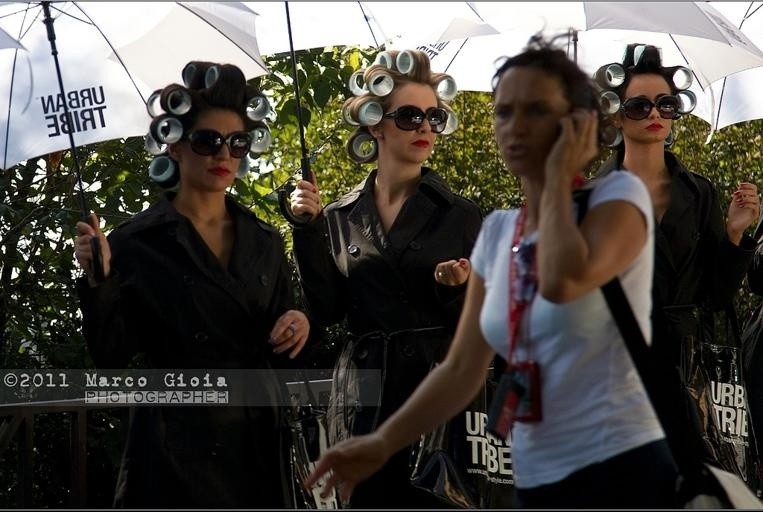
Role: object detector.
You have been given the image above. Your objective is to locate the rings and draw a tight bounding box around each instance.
[288,324,296,337]
[438,271,449,281]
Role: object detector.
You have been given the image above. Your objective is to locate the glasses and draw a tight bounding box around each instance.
[179,126,253,160]
[382,102,448,134]
[615,91,682,122]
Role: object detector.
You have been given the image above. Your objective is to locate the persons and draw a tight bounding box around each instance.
[72,62,309,509]
[305,48,679,508]
[290,50,484,509]
[591,59,759,377]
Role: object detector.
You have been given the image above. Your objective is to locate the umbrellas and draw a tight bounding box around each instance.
[234,0,501,227]
[0,0,269,293]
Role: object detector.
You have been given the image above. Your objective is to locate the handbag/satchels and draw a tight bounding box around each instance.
[405,278,515,509]
[676,462,763,510]
[672,288,763,495]
[276,341,342,509]
